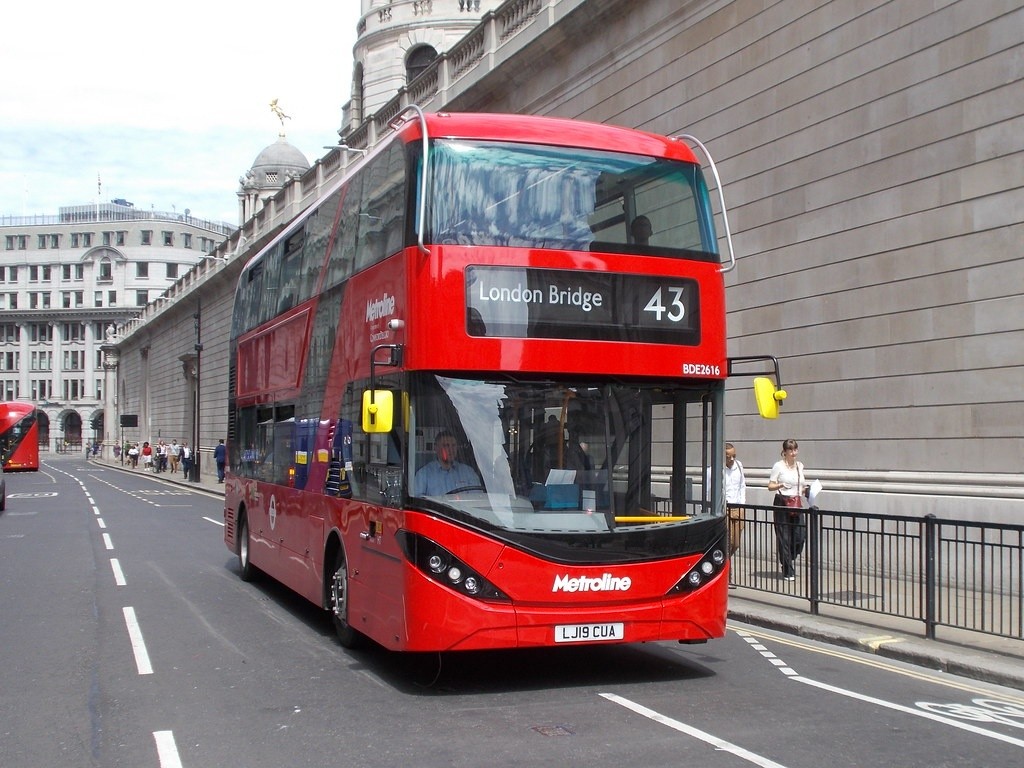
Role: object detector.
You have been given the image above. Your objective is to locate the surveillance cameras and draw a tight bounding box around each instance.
[388,319,398,331]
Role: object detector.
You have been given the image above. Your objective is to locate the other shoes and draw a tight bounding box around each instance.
[782,566,795,580]
[162,469,165,472]
[219,478,224,483]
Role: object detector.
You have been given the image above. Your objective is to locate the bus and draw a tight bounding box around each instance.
[218,103,789,657]
[0,402,39,472]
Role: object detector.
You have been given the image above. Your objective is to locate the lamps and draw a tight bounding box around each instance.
[198,256,227,264]
[129,318,144,323]
[323,145,367,156]
[154,296,174,303]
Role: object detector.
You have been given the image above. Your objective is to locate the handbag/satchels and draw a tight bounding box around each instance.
[782,495,803,517]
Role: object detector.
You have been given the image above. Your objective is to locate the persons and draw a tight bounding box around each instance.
[124,440,131,465]
[214,439,227,484]
[167,439,179,473]
[176,442,192,479]
[140,442,152,471]
[157,440,168,472]
[113,441,121,463]
[629,214,653,246]
[700,444,746,589]
[128,442,140,469]
[769,440,809,581]
[414,431,484,496]
[93,441,99,460]
